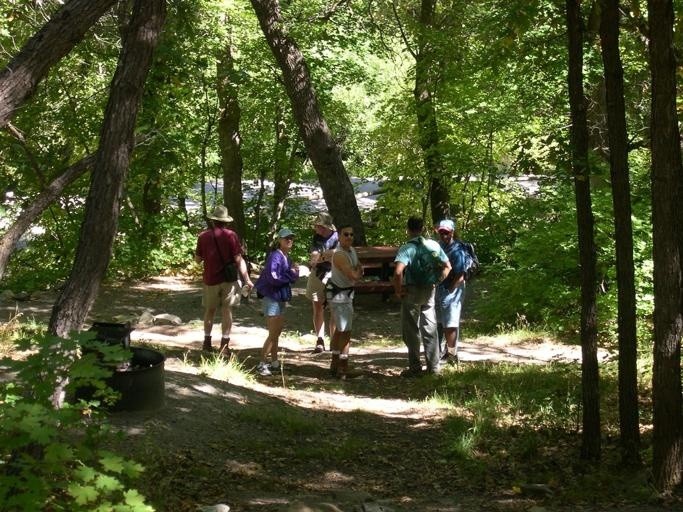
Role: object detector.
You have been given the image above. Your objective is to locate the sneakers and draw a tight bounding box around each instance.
[420,346,441,358]
[440,350,457,366]
[256,361,272,377]
[316,337,325,352]
[401,365,422,379]
[268,362,291,373]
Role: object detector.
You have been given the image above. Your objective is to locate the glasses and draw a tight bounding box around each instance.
[344,233,354,236]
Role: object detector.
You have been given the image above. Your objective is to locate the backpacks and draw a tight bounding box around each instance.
[406,237,448,287]
[458,240,480,280]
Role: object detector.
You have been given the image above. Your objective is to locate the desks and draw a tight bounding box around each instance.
[324,246,399,301]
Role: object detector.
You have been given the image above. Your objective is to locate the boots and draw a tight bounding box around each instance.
[336,357,364,378]
[202,336,213,350]
[330,353,354,374]
[219,337,231,356]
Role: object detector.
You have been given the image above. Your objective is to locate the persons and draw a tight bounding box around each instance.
[391,214,454,378]
[323,223,365,378]
[193,206,254,359]
[251,227,301,376]
[306,214,340,353]
[436,219,467,363]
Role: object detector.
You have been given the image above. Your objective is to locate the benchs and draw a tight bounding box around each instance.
[351,281,395,300]
[316,260,396,277]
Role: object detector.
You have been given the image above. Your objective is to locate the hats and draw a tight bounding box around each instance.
[438,220,455,234]
[278,229,296,237]
[206,206,234,224]
[309,212,338,232]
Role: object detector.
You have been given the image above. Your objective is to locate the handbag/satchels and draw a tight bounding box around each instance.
[224,255,251,283]
[280,271,292,302]
[310,246,325,279]
